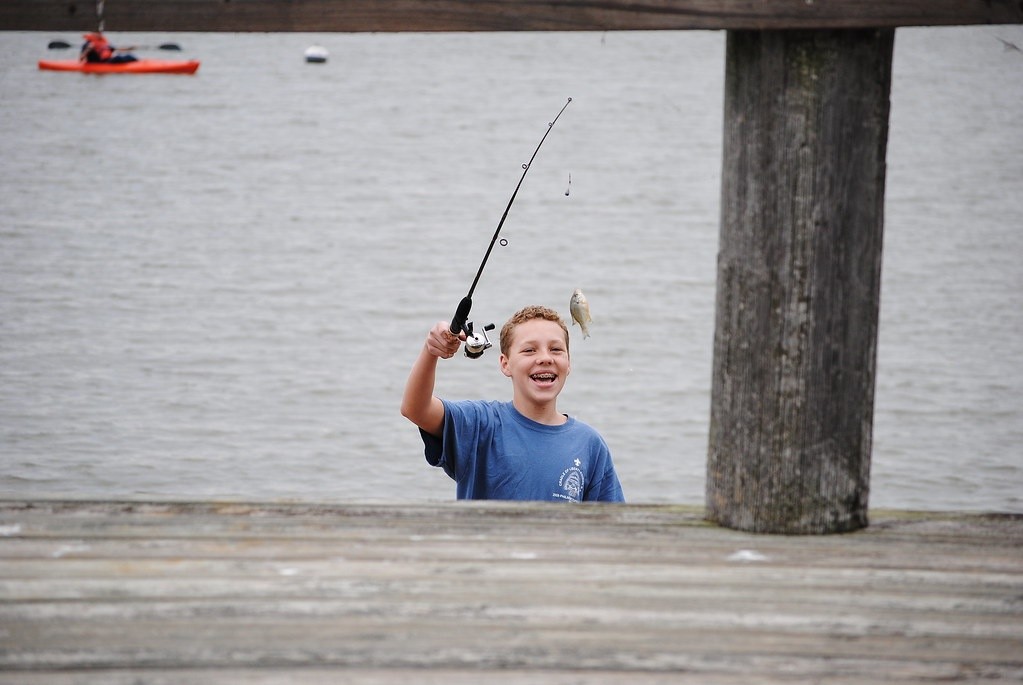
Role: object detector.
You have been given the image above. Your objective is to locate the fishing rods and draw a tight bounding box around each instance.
[449,95,572,360]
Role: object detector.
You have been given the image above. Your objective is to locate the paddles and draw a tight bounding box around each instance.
[46,42,183,51]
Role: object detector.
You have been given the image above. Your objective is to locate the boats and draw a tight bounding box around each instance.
[38,57,200,75]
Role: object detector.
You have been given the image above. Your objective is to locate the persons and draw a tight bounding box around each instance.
[401,306,628,504]
[80,25,138,63]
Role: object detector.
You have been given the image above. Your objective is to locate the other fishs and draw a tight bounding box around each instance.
[570,288,594,341]
